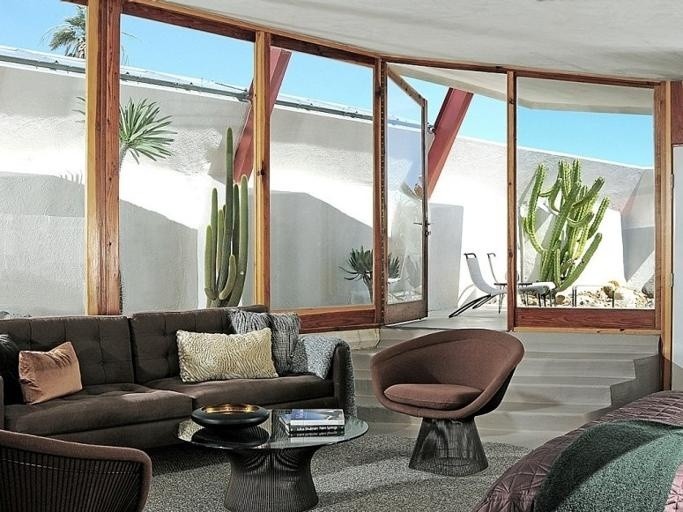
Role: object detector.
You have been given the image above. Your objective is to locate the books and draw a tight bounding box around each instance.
[279,414,345,437]
[290,409,345,431]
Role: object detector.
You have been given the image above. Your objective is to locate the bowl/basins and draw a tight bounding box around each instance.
[189,424,268,447]
[190,402,270,430]
